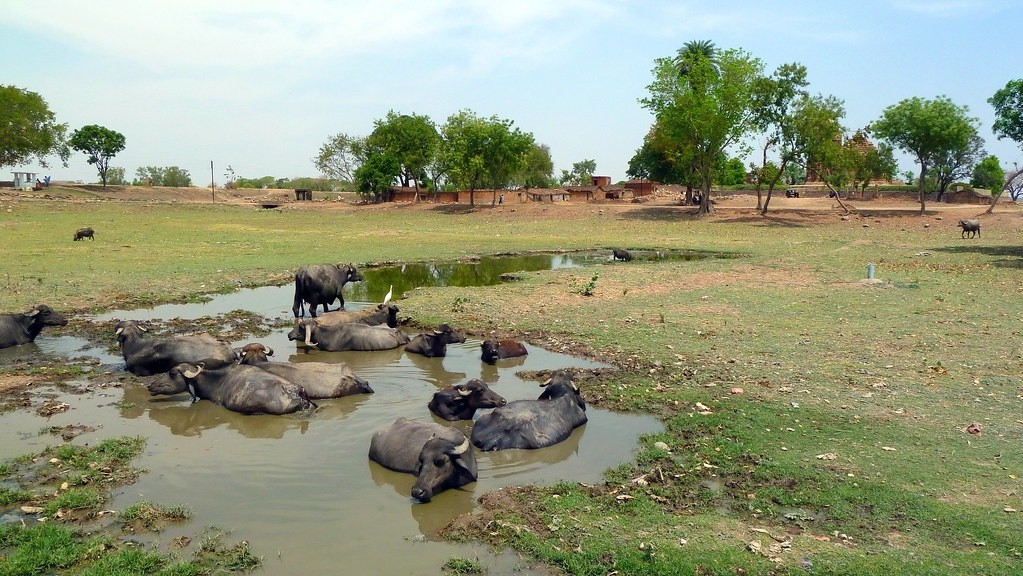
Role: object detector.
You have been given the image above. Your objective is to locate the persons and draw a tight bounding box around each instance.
[499,194,505,205]
[679,190,702,205]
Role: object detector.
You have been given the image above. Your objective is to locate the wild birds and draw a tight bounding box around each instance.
[384,284,393,304]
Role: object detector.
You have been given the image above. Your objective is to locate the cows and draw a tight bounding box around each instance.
[405,351,466,388]
[404,323,466,357]
[470,371,588,451]
[480,338,528,365]
[428,377,507,421]
[73,227,94,241]
[957,219,981,239]
[613,248,631,262]
[149,399,309,439]
[292,262,363,318]
[288,304,410,351]
[368,458,477,542]
[114,319,375,416]
[368,417,478,500]
[480,354,528,383]
[0,304,68,350]
